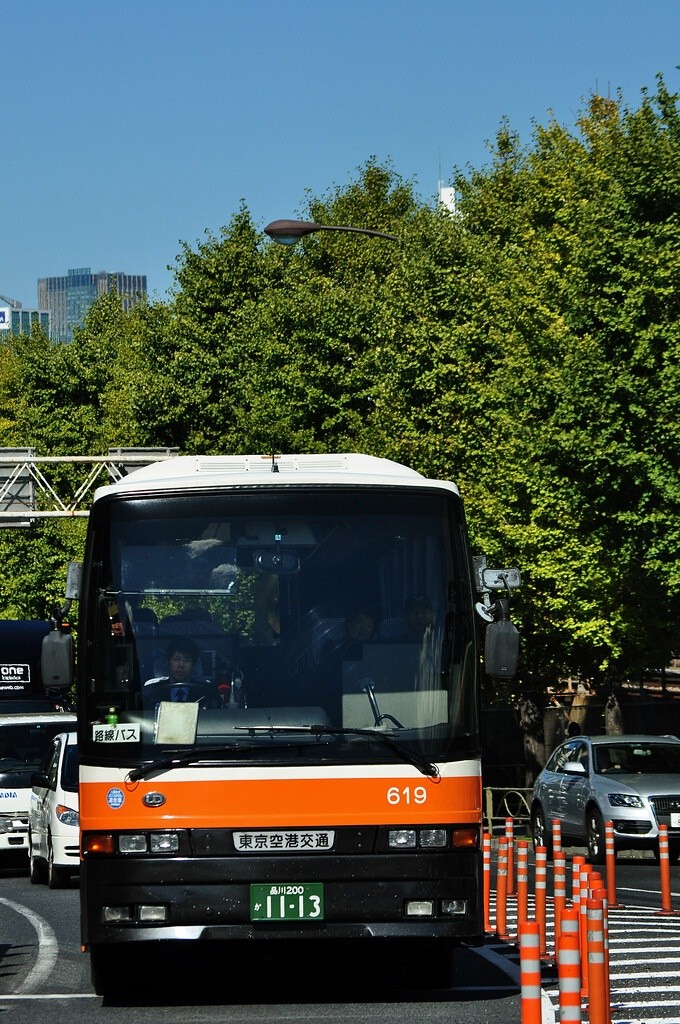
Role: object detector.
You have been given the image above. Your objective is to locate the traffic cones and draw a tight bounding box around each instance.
[482,818,617,1024]
[604,822,623,909]
[654,825,679,914]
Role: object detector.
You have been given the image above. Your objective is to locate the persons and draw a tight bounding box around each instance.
[147,634,223,713]
[404,592,433,636]
[345,609,374,642]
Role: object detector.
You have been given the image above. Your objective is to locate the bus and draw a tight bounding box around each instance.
[67,451,519,1006]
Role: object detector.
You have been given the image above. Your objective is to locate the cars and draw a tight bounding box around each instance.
[530,734,680,865]
[27,732,80,889]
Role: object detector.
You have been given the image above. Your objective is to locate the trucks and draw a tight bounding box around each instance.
[0,711,77,876]
